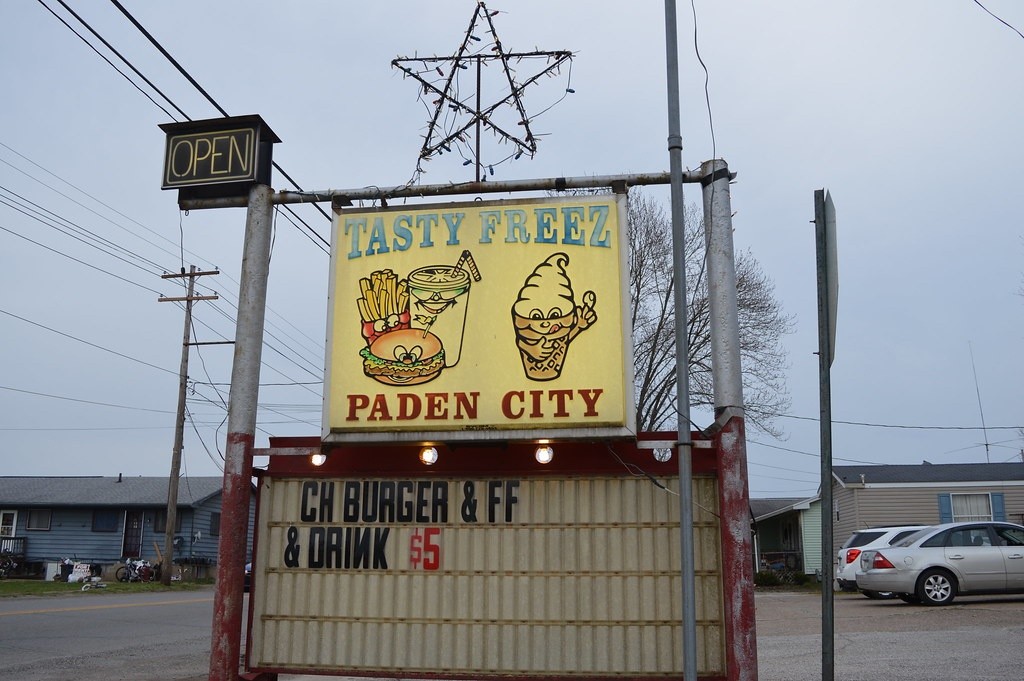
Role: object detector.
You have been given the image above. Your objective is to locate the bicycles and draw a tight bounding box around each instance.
[116,556,156,582]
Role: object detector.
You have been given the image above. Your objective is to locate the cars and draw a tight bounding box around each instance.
[855,521,1024,606]
[243,563,251,593]
[837,522,991,599]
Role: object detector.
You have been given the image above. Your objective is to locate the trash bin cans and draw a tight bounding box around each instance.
[60,564,74,582]
[89,564,102,576]
